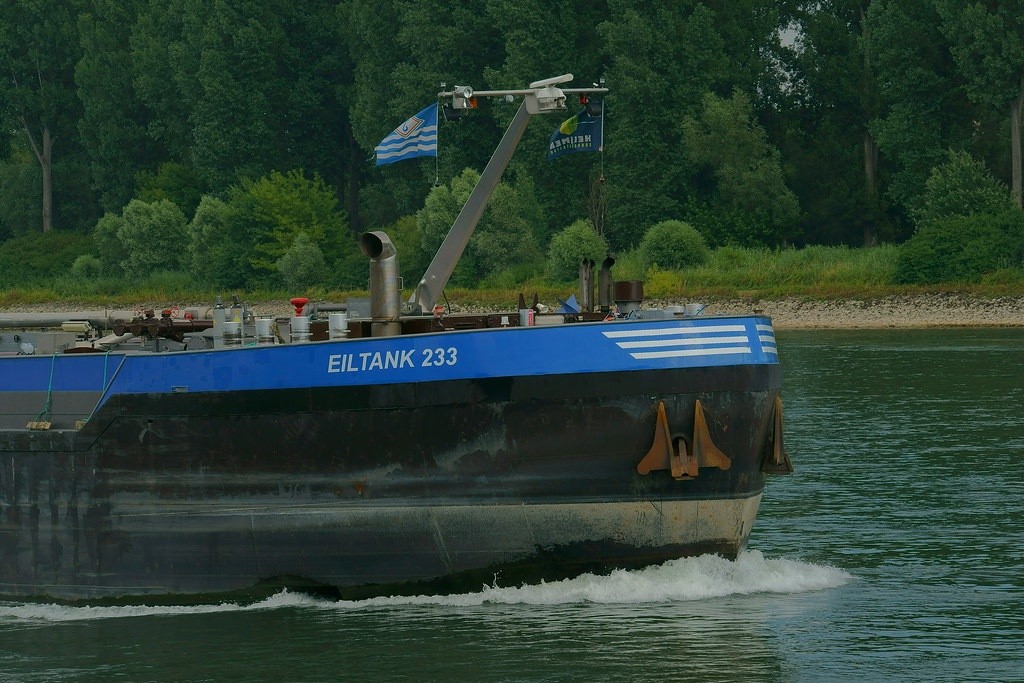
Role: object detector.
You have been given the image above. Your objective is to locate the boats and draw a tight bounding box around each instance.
[0,72,793,607]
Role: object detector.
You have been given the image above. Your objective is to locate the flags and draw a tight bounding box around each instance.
[549,98,602,161]
[374,103,437,165]
